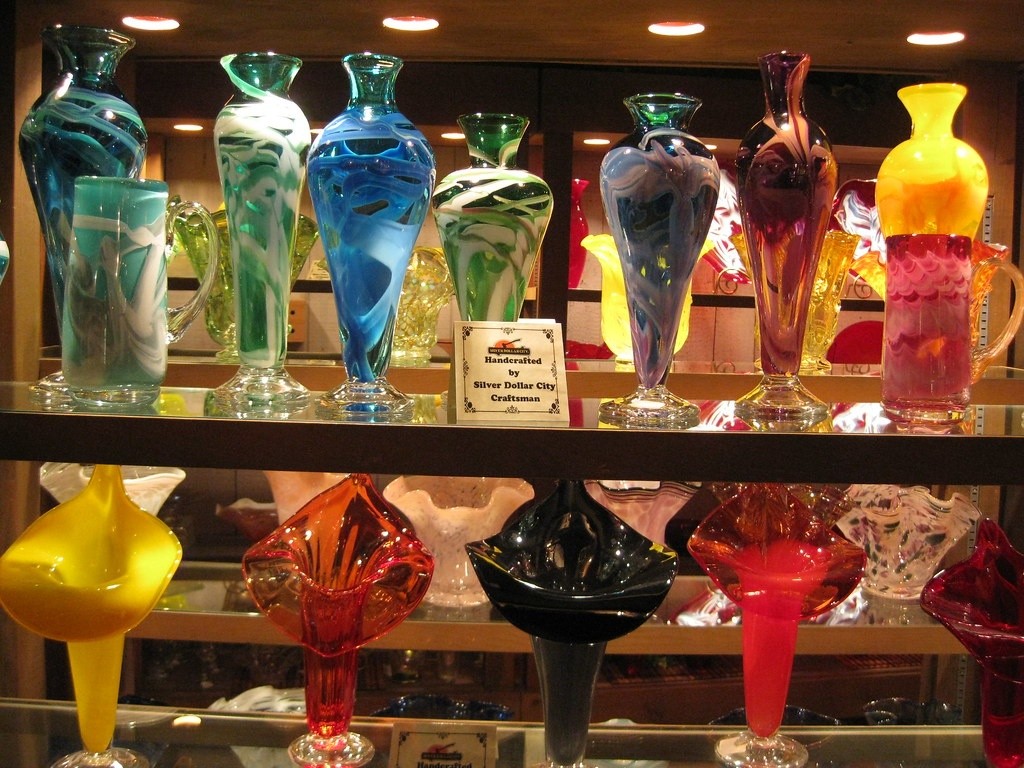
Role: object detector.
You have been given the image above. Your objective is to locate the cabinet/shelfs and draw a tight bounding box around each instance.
[0,380,1024,766]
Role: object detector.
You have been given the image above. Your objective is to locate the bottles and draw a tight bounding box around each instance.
[734,52,838,418]
[212,51,313,405]
[432,112,553,323]
[873,83,990,239]
[599,92,720,429]
[307,52,436,415]
[567,176,593,292]
[18,23,147,399]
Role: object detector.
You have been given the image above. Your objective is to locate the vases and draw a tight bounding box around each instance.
[19,22,989,430]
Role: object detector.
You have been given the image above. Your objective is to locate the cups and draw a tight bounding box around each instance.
[314,241,456,370]
[730,223,864,377]
[61,176,219,409]
[581,225,713,365]
[881,234,1024,426]
[165,194,320,364]
